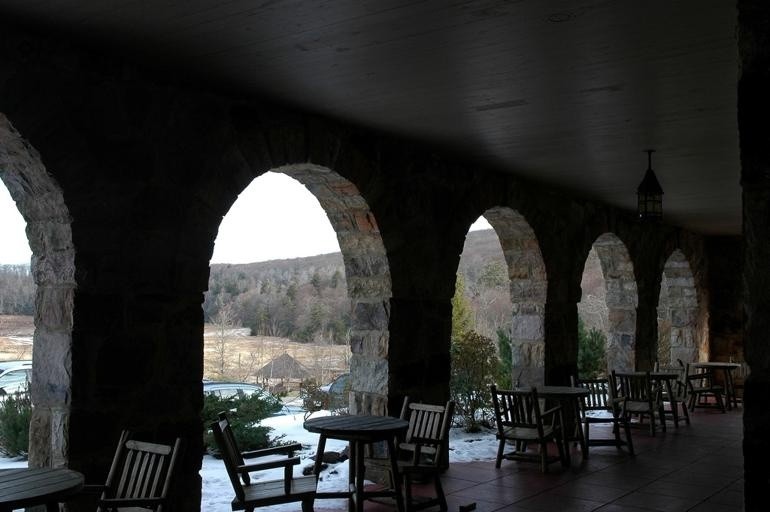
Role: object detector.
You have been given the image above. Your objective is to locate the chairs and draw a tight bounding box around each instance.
[358,395,456,512]
[490,354,752,475]
[74,425,182,512]
[213,411,319,511]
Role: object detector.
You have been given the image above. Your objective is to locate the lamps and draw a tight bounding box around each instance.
[635,150,665,220]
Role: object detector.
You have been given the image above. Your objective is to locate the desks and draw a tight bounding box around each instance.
[303,413,411,512]
[0,465,85,511]
